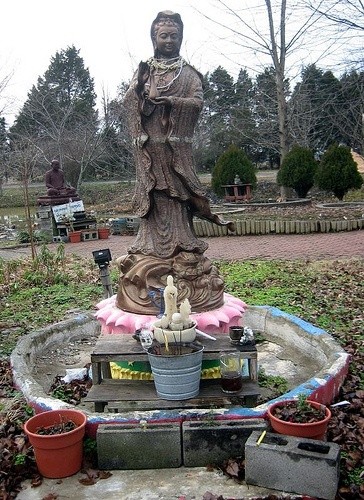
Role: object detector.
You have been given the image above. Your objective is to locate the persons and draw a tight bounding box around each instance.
[44,159,76,195]
[163,274,178,319]
[114,9,236,259]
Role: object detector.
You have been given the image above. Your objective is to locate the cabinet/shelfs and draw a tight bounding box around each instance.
[83,334,261,413]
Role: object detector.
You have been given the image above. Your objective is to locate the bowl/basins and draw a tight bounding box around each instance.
[153,319,198,344]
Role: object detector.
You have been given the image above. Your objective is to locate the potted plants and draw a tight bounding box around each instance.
[56,198,82,243]
[88,200,110,239]
[267,393,332,440]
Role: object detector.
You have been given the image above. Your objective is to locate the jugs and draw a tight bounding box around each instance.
[218,353,243,394]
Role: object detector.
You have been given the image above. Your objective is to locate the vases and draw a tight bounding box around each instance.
[24,409,87,479]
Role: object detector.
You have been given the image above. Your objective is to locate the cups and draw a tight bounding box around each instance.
[229,325,244,344]
[140,331,154,351]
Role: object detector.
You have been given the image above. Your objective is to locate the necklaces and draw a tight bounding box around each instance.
[143,56,187,93]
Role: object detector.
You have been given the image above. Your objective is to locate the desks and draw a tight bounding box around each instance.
[221,184,252,202]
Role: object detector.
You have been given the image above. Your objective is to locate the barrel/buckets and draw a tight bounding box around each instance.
[145,342,205,401]
